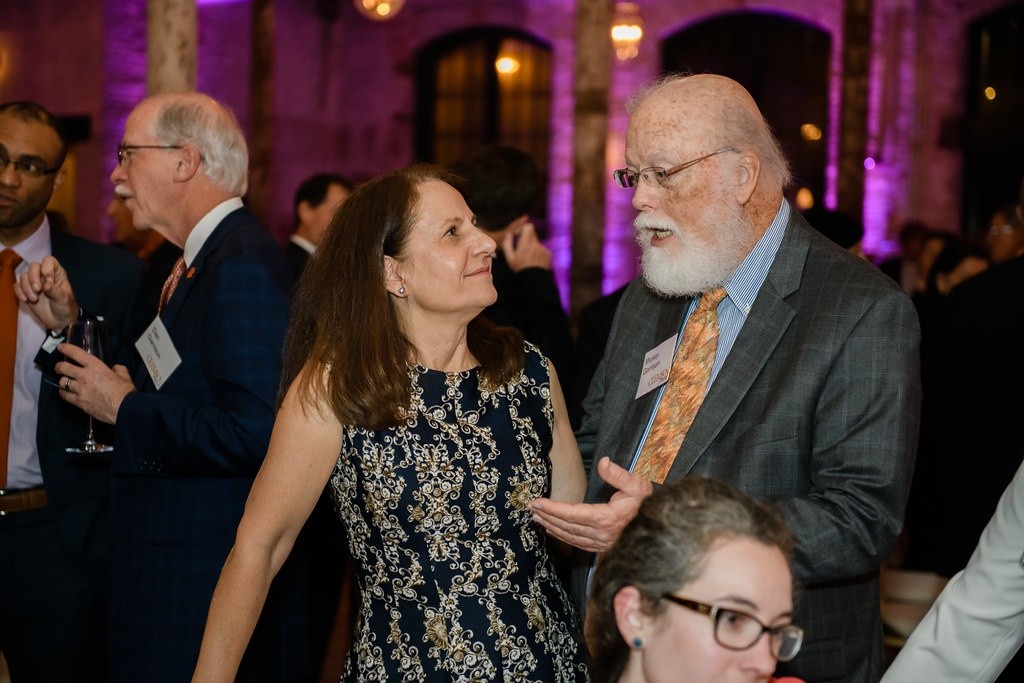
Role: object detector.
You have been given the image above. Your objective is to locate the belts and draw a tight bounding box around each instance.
[0,490,49,516]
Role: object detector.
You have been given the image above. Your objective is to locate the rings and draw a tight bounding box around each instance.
[65,379,71,391]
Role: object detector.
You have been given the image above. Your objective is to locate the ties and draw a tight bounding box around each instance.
[156,257,188,319]
[0,249,23,488]
[593,285,728,567]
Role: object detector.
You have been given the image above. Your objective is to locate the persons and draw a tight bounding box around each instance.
[527,74,920,683]
[817,192,1024,683]
[0,90,368,683]
[191,161,590,683]
[583,478,805,683]
[453,148,631,445]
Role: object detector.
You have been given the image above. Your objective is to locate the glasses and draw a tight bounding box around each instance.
[612,146,743,189]
[659,591,803,662]
[0,157,57,178]
[117,143,204,166]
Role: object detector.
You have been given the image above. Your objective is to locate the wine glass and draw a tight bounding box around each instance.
[61,318,115,453]
